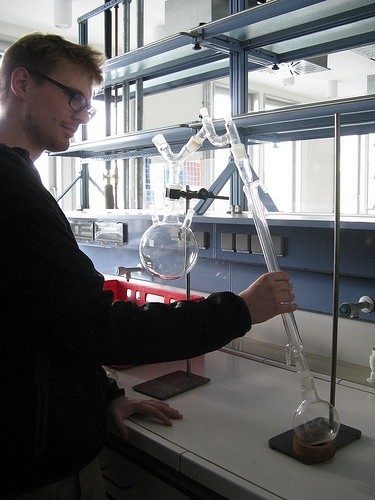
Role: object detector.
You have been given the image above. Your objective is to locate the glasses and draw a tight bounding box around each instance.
[31,71,96,119]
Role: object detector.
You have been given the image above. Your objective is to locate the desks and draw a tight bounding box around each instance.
[102,272,375,500]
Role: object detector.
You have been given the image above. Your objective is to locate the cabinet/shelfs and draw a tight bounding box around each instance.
[48,0,375,230]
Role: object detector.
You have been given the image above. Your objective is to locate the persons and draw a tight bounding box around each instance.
[0,31,298,500]
